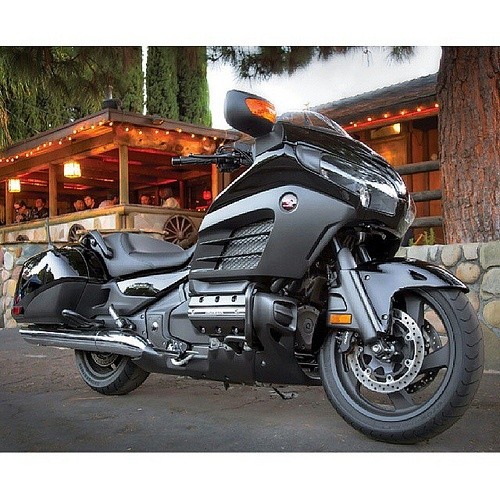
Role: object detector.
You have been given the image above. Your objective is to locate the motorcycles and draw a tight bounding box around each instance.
[11,90,484,444]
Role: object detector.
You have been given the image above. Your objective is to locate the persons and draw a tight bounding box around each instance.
[73,199,84,211]
[99,193,119,207]
[159,188,180,209]
[31,198,49,220]
[84,195,98,209]
[14,200,33,222]
[140,193,152,205]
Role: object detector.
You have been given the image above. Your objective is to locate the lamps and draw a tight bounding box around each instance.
[63,162,81,179]
[8,177,21,192]
[150,115,165,125]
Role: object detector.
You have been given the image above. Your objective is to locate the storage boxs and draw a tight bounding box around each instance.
[11,247,109,325]
